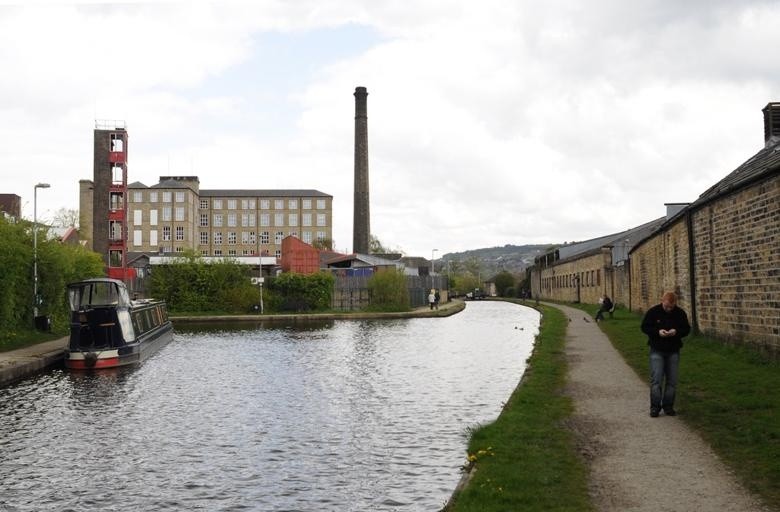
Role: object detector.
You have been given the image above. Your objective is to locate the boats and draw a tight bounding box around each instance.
[63,278,175,372]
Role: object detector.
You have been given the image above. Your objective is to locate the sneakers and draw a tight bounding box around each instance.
[664,409,675,416]
[650,409,659,417]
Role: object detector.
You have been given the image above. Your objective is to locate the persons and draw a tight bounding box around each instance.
[640,290,691,418]
[84,304,100,346]
[595,293,613,322]
[434,290,440,310]
[427,291,436,310]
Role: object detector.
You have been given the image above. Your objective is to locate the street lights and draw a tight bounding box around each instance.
[431,249,439,288]
[478,272,483,284]
[31,182,50,320]
[447,260,453,290]
[258,234,269,314]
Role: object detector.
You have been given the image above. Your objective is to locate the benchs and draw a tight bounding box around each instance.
[608,303,616,318]
